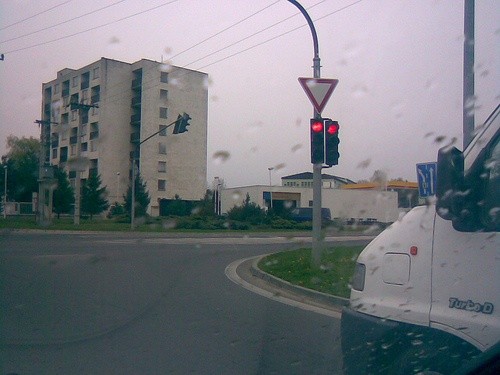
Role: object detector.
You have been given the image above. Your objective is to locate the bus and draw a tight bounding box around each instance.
[340,101,499,374]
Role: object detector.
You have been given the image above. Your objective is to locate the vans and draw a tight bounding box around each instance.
[288,208,331,223]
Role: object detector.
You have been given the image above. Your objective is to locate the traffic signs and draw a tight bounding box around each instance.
[416,162,438,199]
[298,77,339,114]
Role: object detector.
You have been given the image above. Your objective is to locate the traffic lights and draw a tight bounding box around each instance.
[310,119,324,163]
[325,121,338,165]
[179,112,192,133]
[173,113,183,134]
